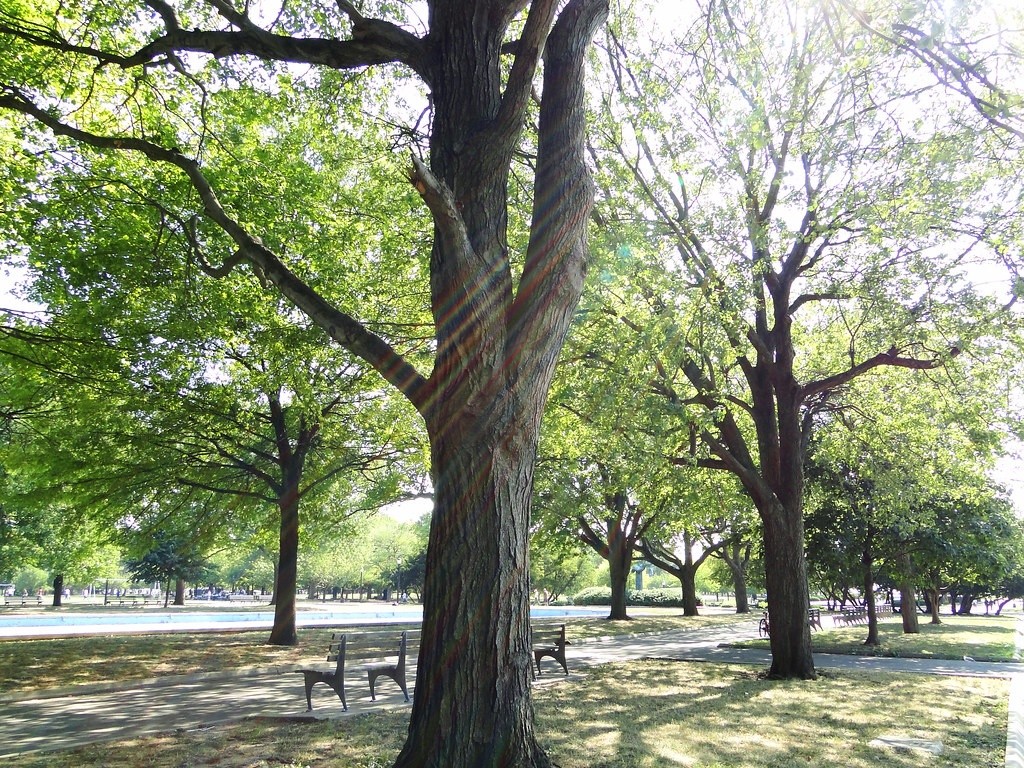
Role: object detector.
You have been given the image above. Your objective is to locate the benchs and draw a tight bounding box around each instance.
[294,629,421,712]
[759,608,822,637]
[532,624,570,677]
[876,604,893,621]
[833,606,869,628]
